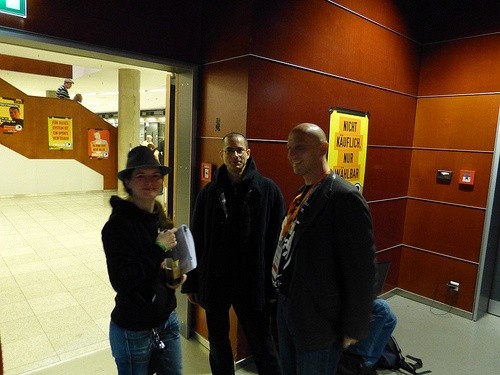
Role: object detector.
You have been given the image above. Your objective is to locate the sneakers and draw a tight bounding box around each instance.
[339,348,377,375]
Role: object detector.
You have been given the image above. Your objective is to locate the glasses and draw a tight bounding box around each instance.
[222,148,247,153]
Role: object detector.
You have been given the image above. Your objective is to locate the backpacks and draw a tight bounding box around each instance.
[374,334,432,375]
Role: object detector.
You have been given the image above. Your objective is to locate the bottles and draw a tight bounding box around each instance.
[164,230,181,283]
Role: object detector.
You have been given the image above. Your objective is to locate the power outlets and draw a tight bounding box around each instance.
[449,281,460,292]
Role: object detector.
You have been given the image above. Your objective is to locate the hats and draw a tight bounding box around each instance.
[116,146,170,182]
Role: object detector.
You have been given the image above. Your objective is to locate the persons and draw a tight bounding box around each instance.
[142,133,166,195]
[56,79,83,105]
[102,146,187,374]
[268,123,376,375]
[0,107,25,131]
[180,132,285,375]
[339,299,397,375]
